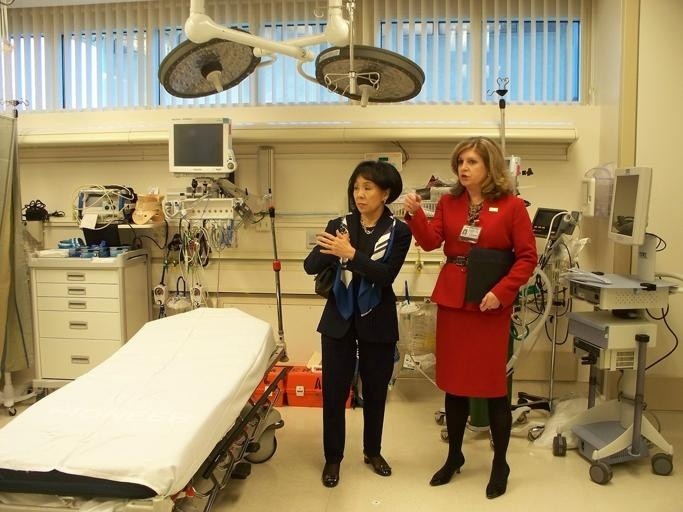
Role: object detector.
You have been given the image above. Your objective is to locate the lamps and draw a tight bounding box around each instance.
[157,0,422,107]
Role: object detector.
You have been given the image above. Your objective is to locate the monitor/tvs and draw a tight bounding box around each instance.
[606,166,653,246]
[168,117,237,175]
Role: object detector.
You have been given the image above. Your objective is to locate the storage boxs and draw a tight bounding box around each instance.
[250,365,355,410]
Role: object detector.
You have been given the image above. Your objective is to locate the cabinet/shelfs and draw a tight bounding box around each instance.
[28,246,149,403]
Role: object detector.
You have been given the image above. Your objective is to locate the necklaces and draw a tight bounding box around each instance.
[360,216,374,235]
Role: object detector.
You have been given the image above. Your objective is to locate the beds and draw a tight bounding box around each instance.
[2,307,283,511]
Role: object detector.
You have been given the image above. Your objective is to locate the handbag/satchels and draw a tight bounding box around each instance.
[464,246,521,306]
[315,263,337,299]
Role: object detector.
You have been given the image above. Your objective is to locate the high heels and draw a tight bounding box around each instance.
[486,458,510,498]
[430,453,465,486]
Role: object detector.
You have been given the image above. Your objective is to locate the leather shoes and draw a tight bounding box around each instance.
[322,459,340,487]
[364,454,391,477]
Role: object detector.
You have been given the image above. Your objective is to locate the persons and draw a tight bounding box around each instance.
[301,160,413,488]
[401,134,539,501]
